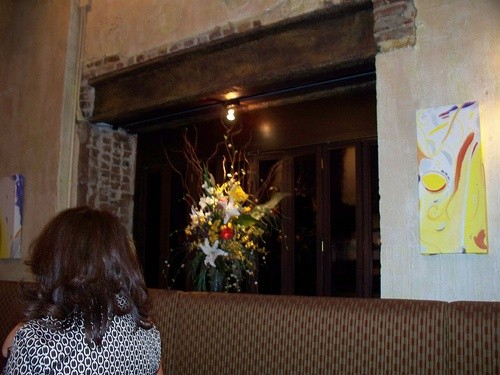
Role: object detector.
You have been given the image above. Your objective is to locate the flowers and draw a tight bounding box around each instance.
[162,117,304,295]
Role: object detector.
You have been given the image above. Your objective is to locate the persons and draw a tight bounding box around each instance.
[0,207,167,375]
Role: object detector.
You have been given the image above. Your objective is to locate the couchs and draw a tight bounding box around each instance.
[0,280,500,375]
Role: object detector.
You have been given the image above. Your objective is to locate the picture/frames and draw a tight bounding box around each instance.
[0,175,23,261]
[415,101,489,256]
